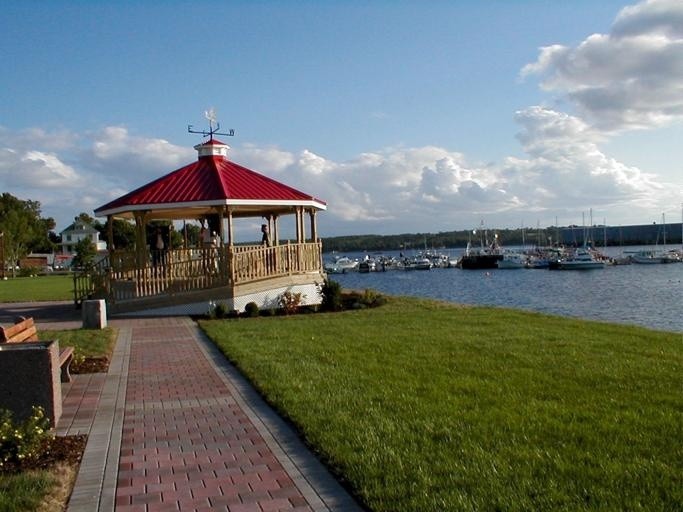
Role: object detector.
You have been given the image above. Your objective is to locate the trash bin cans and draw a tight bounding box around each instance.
[83,299,106,329]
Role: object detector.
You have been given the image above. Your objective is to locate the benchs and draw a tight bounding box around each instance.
[0,315,75,382]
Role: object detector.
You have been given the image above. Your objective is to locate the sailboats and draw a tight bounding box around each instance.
[458,207,683,271]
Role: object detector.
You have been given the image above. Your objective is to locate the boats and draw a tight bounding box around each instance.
[323,250,458,274]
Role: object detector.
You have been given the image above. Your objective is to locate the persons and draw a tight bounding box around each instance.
[150,224,272,278]
